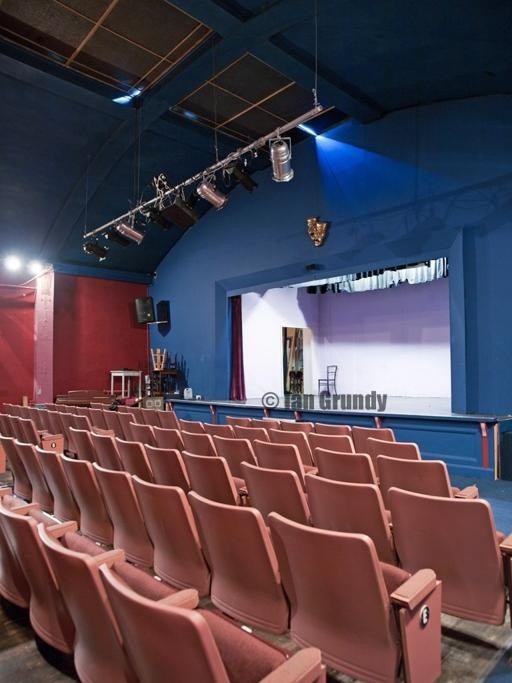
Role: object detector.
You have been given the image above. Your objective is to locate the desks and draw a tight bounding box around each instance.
[108,369,144,397]
[152,370,179,394]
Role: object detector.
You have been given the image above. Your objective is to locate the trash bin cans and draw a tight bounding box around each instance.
[142,397,164,411]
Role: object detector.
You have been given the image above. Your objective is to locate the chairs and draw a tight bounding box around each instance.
[0,394,512,682]
[316,364,338,395]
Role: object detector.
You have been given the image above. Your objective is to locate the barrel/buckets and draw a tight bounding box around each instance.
[183,387,192,400]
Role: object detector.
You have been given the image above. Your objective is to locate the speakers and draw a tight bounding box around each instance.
[135,296,155,323]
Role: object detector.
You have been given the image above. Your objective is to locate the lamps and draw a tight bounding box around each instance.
[79,0,325,261]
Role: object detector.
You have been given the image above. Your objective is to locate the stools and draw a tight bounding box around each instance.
[147,347,169,370]
